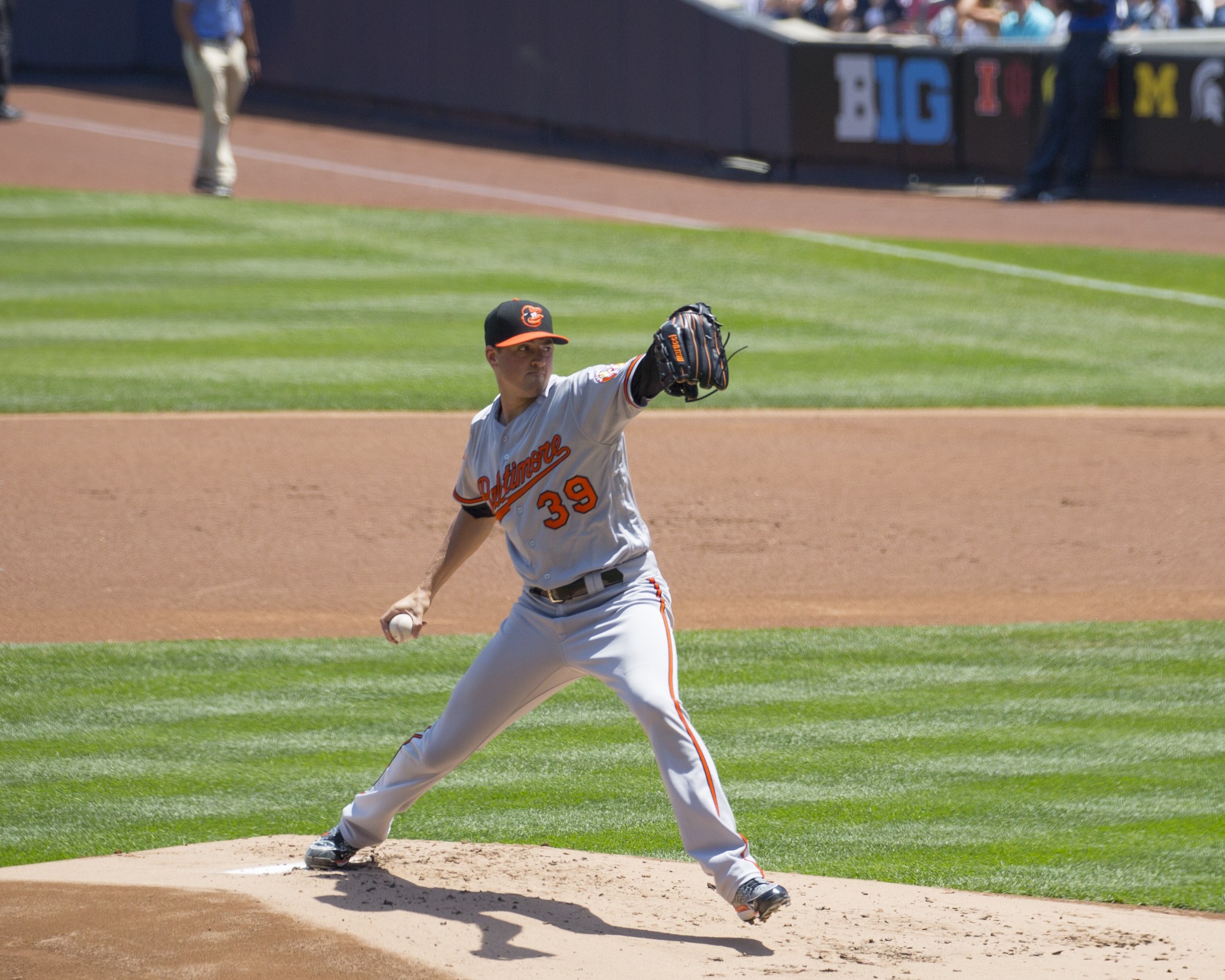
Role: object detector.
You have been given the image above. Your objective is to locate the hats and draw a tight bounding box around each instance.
[484,298,569,347]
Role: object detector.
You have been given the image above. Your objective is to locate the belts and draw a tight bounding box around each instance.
[529,568,623,604]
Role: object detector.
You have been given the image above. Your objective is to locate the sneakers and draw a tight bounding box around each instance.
[732,877,791,924]
[304,822,359,871]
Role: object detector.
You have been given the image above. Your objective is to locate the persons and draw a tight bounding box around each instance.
[173,0,261,198]
[304,300,792,924]
[744,0,1225,202]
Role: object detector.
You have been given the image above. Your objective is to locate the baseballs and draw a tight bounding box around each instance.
[388,613,413,643]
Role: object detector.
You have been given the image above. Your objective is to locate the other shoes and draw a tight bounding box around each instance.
[213,185,232,198]
[195,178,215,191]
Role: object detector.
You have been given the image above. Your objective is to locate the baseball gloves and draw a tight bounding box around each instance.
[652,303,730,402]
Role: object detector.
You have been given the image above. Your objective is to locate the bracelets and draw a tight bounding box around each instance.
[248,53,260,60]
